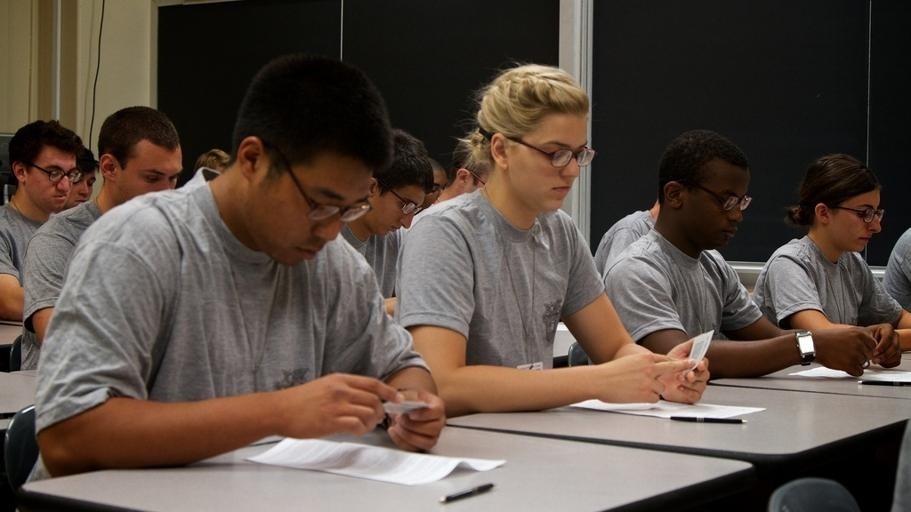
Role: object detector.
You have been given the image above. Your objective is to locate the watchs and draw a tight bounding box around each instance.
[794,329,817,366]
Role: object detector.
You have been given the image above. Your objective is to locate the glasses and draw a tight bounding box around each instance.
[31,164,82,183]
[689,183,751,210]
[390,189,423,215]
[280,155,373,224]
[831,206,885,224]
[502,134,596,167]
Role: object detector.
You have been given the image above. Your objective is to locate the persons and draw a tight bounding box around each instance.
[752,154,911,357]
[2,105,493,367]
[400,63,710,418]
[588,198,662,366]
[602,129,878,377]
[32,54,448,475]
[883,228,911,312]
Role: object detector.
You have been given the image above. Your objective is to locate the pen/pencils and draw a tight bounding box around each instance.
[671,416,748,424]
[442,483,495,502]
[858,379,910,387]
